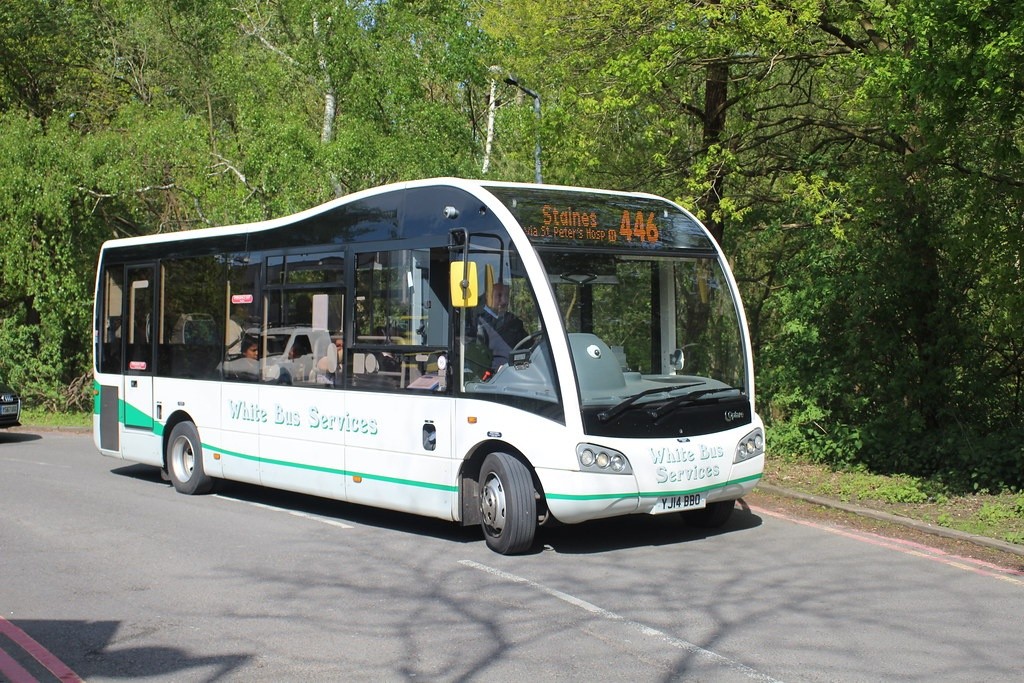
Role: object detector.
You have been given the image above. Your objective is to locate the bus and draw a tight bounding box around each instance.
[91,177,765,556]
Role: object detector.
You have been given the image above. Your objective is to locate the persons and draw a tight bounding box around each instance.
[240,332,258,359]
[476,283,534,373]
[331,332,344,351]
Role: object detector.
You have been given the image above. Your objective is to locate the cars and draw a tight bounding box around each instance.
[0,381,22,428]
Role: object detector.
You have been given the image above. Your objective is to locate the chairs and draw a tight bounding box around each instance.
[455,305,479,338]
[146,310,222,347]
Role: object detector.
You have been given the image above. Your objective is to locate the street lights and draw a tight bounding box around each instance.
[504,74,544,184]
